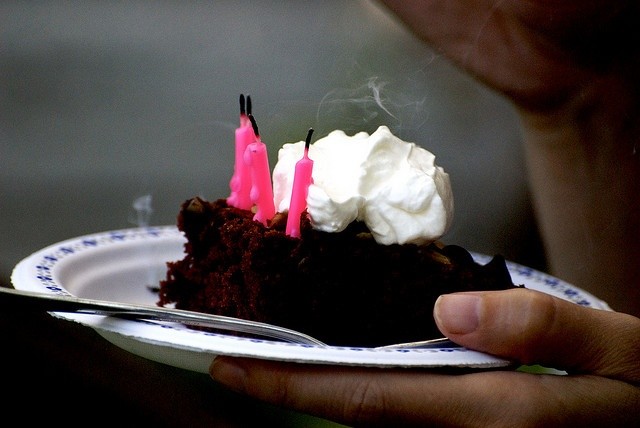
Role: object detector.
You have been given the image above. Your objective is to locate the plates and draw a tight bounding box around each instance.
[9,226,614,379]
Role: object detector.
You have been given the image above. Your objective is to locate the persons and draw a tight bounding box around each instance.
[208,1,640,428]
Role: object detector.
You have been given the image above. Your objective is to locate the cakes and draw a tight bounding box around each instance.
[151,125,526,349]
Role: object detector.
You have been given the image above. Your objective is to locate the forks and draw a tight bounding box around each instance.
[0,284,322,343]
